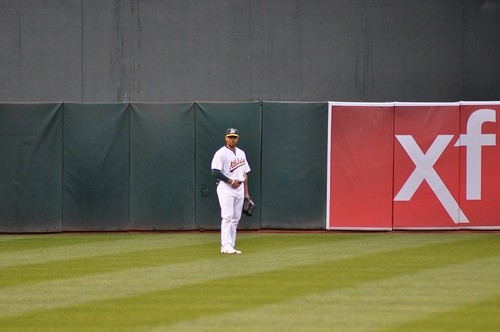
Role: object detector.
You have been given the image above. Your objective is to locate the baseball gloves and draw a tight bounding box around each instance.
[242,198,256,217]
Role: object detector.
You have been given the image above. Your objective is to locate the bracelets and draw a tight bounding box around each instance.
[230,180,234,186]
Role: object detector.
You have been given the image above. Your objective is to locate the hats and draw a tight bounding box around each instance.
[226,128,238,136]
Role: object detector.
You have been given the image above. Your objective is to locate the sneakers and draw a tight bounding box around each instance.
[221,244,241,254]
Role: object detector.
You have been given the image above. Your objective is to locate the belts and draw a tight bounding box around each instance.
[240,181,245,183]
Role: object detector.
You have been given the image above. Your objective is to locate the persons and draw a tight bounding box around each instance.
[210,127,251,254]
